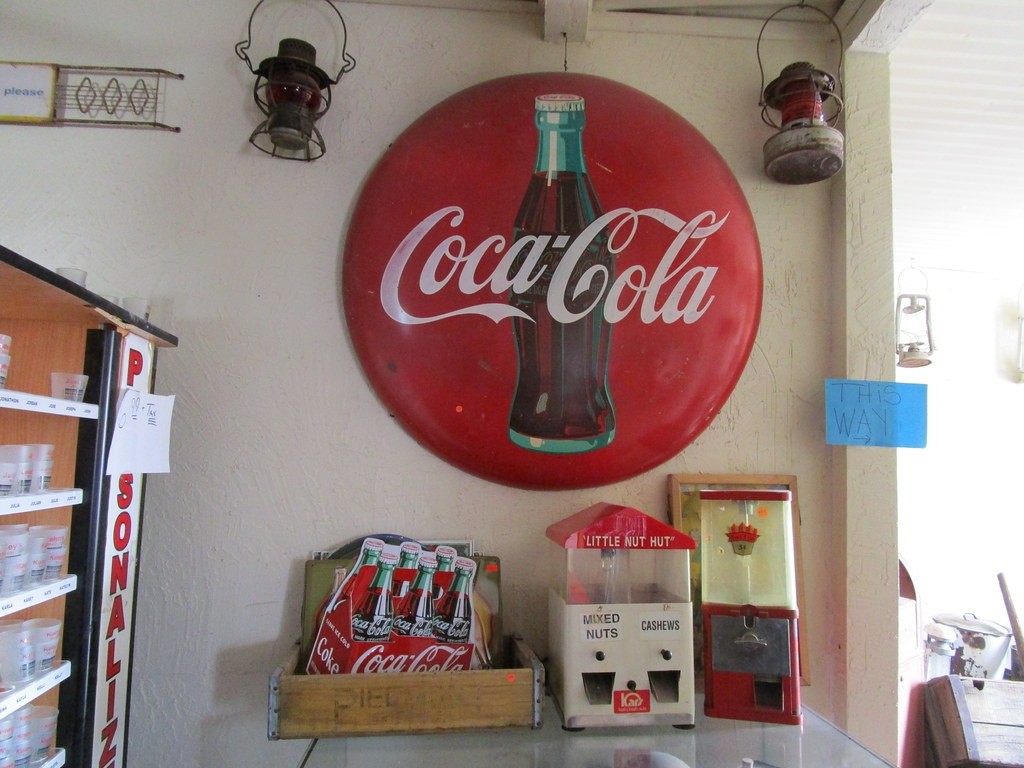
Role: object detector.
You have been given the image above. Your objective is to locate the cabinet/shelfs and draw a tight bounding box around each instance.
[0,245,179,768]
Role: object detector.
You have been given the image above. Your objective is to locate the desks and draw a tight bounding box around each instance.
[295,687,897,768]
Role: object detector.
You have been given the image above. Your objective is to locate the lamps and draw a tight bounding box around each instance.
[756,4,845,184]
[895,256,935,368]
[234,0,356,163]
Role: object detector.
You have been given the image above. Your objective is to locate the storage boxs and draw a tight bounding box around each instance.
[266,635,543,740]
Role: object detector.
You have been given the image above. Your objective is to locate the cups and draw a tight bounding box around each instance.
[1,354,12,388]
[51,370,88,402]
[57,267,153,322]
[1,334,13,355]
[0,443,59,768]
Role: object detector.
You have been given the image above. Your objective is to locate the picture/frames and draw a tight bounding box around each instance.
[666,473,811,694]
